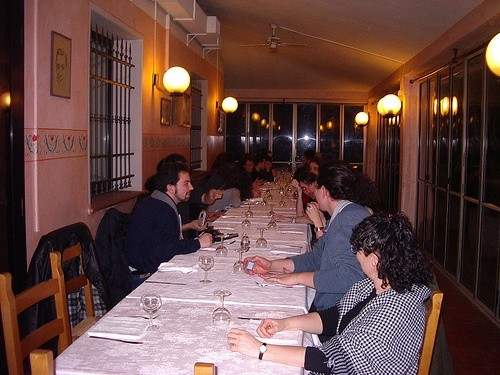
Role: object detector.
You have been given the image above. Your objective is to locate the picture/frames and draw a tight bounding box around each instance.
[182,94,192,127]
[161,98,170,125]
[51,30,73,98]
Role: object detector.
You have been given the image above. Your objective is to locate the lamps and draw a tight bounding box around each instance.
[486,32,500,76]
[163,66,190,95]
[219,96,238,113]
[354,111,369,126]
[377,94,402,118]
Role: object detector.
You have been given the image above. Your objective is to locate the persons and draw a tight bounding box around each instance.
[291,148,331,241]
[165,154,222,238]
[226,211,434,375]
[243,158,374,311]
[199,150,273,214]
[122,158,213,290]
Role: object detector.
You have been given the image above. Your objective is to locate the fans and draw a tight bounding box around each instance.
[238,23,308,49]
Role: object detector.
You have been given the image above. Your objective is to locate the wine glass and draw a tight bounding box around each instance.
[242,213,252,229]
[141,293,162,331]
[245,199,254,217]
[198,255,214,284]
[267,212,278,231]
[256,227,267,248]
[263,171,298,206]
[266,203,276,219]
[211,290,233,328]
[233,248,246,274]
[215,235,228,257]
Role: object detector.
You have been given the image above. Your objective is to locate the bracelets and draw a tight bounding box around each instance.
[313,226,325,232]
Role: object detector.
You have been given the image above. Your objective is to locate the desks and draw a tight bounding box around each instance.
[51,181,324,375]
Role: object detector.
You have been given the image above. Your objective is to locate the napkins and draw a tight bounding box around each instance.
[201,244,219,250]
[213,223,237,230]
[113,316,164,327]
[158,262,197,274]
[279,227,304,234]
[270,245,302,255]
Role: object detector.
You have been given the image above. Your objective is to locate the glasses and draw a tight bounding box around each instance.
[351,244,363,255]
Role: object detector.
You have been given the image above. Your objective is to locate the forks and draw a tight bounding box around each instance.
[114,314,160,320]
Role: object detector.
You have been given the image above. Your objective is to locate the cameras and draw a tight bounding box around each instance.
[198,211,207,226]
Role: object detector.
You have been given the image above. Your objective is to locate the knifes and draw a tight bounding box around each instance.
[145,280,187,286]
[89,335,143,345]
[237,316,265,321]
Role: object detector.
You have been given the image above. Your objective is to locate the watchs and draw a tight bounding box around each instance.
[258,343,267,360]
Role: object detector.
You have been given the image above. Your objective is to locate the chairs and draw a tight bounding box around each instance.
[94,208,130,306]
[416,270,444,375]
[22,222,113,344]
[0,251,71,375]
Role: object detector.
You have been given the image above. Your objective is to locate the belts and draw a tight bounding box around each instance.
[132,273,151,279]
